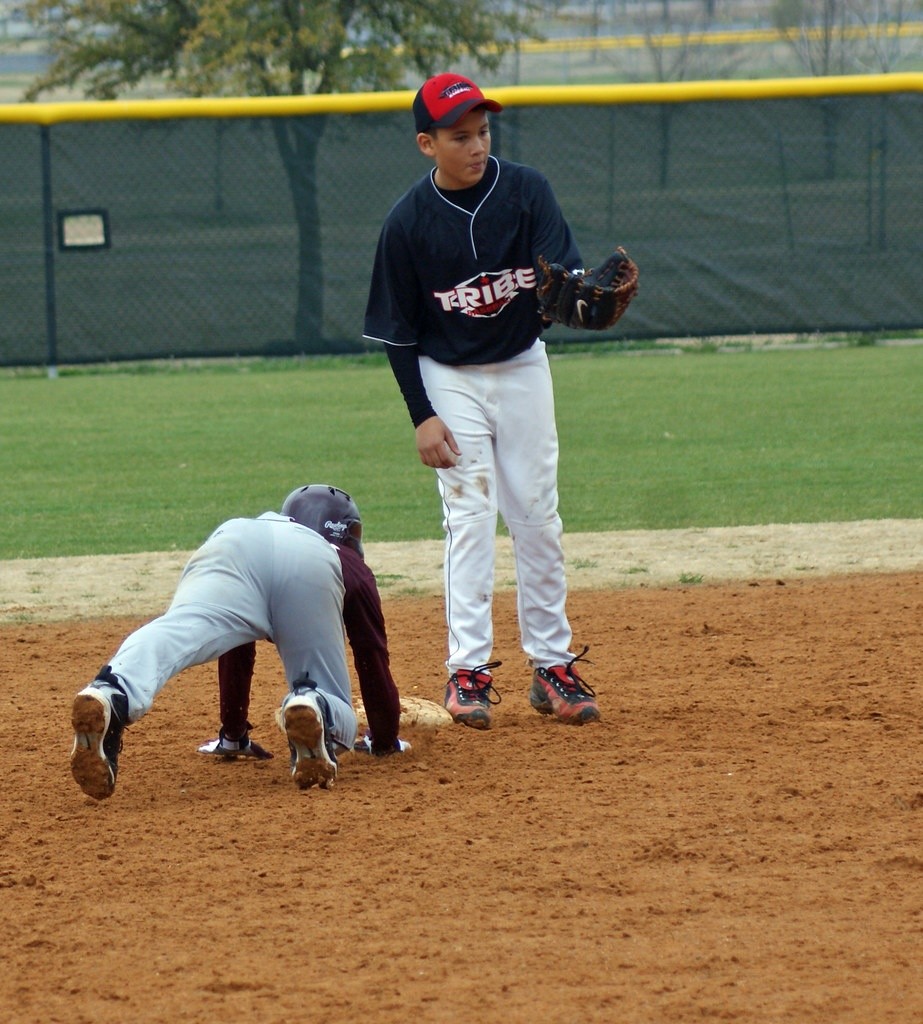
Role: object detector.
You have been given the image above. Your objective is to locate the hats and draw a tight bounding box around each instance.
[413,74,503,134]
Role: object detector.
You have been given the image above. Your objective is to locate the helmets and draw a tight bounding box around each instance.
[281,484,366,555]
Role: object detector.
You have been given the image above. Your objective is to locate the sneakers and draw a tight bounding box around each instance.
[68,665,130,800]
[530,644,600,724]
[444,662,501,728]
[282,679,338,791]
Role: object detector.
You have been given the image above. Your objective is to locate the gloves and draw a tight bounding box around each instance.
[198,737,273,759]
[356,736,412,754]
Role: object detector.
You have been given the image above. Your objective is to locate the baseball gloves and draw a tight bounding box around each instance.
[534,242,642,333]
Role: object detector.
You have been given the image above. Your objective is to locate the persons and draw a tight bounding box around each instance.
[70,485,413,800]
[362,74,639,728]
